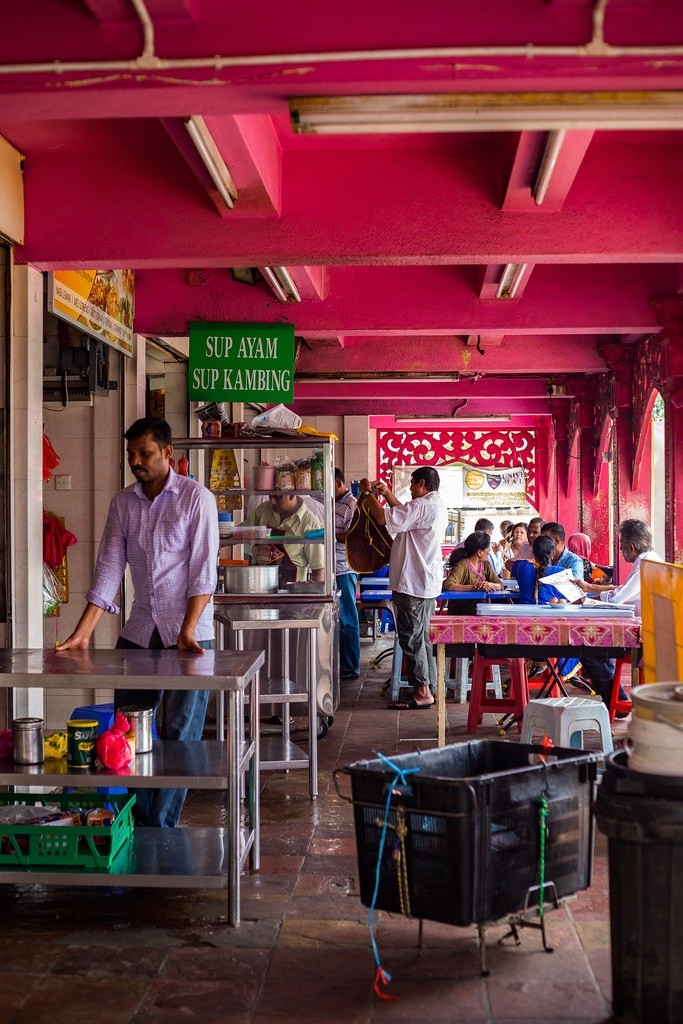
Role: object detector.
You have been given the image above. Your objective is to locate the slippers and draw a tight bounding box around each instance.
[388,693,432,710]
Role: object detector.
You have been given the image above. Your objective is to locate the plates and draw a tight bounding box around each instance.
[546,601,571,608]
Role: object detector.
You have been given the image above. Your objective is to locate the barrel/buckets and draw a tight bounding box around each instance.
[592,680,683,1024]
[251,465,277,491]
[223,565,282,621]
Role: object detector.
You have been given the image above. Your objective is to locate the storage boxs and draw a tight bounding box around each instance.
[0,792,137,870]
[337,737,609,928]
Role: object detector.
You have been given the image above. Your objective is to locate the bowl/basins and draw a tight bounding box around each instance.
[286,580,324,593]
[218,512,234,538]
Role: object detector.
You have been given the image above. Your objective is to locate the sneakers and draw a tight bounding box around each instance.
[614,711,632,721]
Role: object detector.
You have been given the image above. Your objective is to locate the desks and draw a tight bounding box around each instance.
[358,575,521,671]
[429,616,643,747]
[475,602,636,731]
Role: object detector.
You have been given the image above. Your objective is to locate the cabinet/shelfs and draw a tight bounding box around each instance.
[211,607,319,802]
[0,648,265,928]
[170,436,341,604]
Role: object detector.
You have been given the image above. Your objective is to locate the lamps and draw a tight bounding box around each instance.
[181,114,239,210]
[394,414,511,422]
[286,90,683,135]
[292,372,460,383]
[495,263,529,299]
[529,126,567,206]
[231,266,301,303]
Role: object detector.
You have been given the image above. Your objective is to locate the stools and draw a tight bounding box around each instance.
[65,703,158,826]
[380,607,633,776]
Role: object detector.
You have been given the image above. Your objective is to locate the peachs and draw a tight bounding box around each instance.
[549,597,567,604]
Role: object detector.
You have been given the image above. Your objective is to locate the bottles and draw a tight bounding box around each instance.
[178,453,188,477]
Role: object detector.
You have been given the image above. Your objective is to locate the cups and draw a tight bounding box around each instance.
[117,705,153,755]
[10,717,45,764]
[503,569,510,580]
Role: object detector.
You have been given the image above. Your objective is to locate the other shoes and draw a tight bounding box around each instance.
[340,671,360,681]
[528,665,548,678]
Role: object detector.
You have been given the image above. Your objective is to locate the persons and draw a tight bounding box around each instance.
[53,417,220,827]
[442,517,611,684]
[234,494,324,590]
[570,518,664,717]
[334,467,361,680]
[358,466,449,709]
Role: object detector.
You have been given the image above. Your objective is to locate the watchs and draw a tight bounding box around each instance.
[363,490,372,499]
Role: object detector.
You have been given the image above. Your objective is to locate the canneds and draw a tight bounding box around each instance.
[66,719,99,769]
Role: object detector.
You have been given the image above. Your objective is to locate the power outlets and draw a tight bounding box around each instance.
[547,385,556,396]
